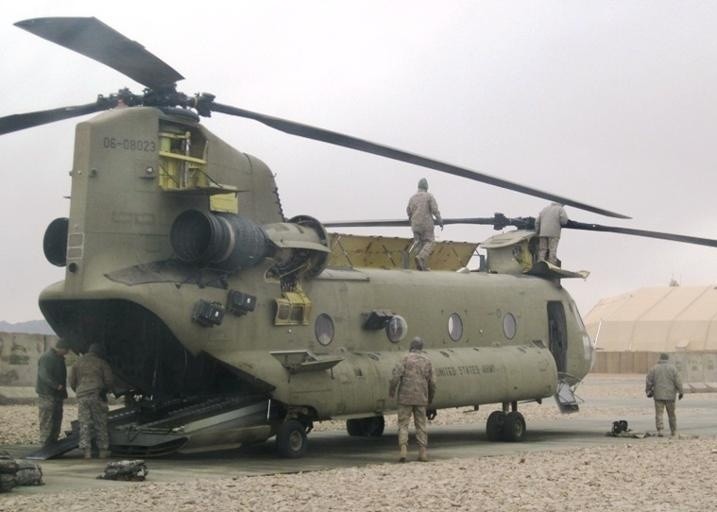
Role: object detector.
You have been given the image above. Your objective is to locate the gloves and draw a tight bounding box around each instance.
[678,393,682,400]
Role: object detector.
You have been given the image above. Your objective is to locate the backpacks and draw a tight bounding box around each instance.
[15,458,42,486]
[95,459,148,482]
[0,451,19,492]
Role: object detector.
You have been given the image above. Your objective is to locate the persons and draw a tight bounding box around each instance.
[68,344,113,457]
[645,352,683,436]
[34,338,70,447]
[388,336,435,462]
[535,202,567,265]
[406,178,443,270]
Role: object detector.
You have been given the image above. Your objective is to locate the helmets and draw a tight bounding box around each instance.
[410,337,422,349]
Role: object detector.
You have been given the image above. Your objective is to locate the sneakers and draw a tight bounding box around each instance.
[423,265,430,271]
[414,256,424,271]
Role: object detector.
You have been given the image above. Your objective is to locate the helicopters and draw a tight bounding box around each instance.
[0,17,717,461]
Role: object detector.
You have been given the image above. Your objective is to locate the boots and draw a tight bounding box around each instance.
[84,448,92,459]
[400,445,407,462]
[98,449,111,458]
[418,447,428,462]
[658,429,664,436]
